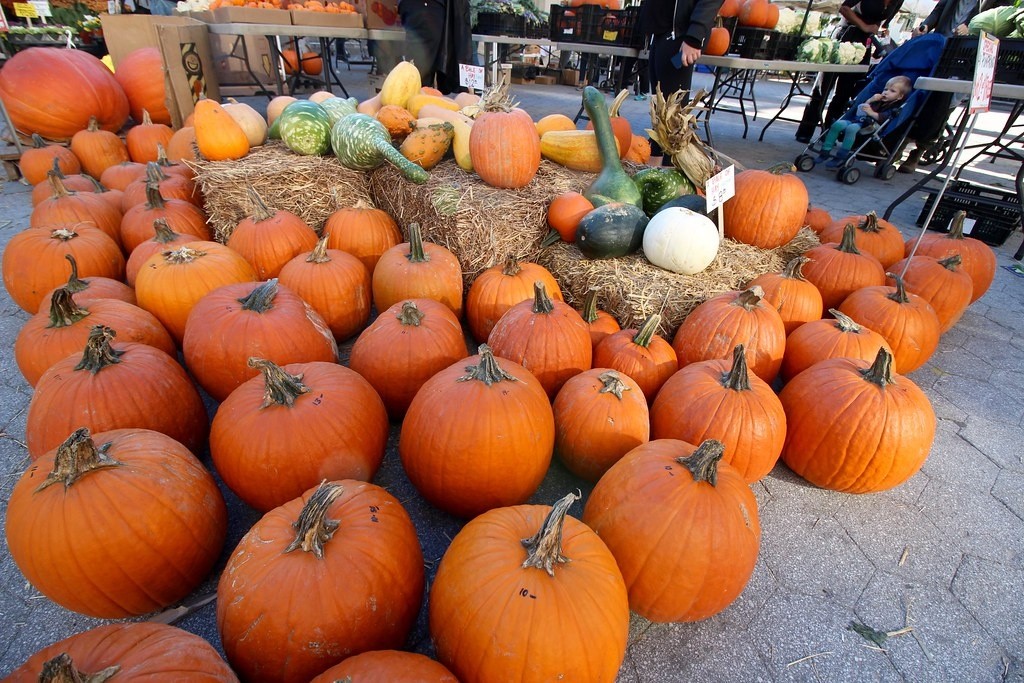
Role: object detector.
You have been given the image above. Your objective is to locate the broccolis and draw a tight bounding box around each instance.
[777,7,866,66]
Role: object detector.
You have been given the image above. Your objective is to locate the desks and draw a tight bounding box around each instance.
[206,23,1024,261]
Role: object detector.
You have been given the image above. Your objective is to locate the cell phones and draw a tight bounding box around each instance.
[672,51,683,69]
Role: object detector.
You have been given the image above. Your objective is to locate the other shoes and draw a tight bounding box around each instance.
[896,149,920,173]
[796,135,811,144]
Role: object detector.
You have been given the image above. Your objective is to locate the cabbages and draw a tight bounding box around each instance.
[968,4,1024,40]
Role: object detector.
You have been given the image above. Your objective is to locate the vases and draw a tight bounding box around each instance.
[94,27,103,36]
[31,33,42,40]
[47,32,59,40]
[66,34,75,40]
[15,33,25,41]
[78,29,94,44]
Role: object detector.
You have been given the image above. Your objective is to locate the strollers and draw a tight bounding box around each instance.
[793,25,956,185]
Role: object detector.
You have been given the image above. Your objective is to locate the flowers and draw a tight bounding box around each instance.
[8,13,102,35]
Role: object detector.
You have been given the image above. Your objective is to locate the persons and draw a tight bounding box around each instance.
[794,0,904,144]
[559,0,649,100]
[813,76,911,167]
[639,0,726,165]
[398,0,472,95]
[897,0,1008,174]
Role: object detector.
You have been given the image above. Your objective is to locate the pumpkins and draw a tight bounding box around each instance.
[554,0,629,40]
[176,0,358,15]
[0,0,132,11]
[0,49,995,683]
[702,0,781,57]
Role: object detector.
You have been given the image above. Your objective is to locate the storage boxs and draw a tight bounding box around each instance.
[720,16,826,61]
[470,12,580,86]
[932,34,1024,87]
[171,0,404,84]
[549,4,641,48]
[100,14,223,107]
[915,179,1023,248]
